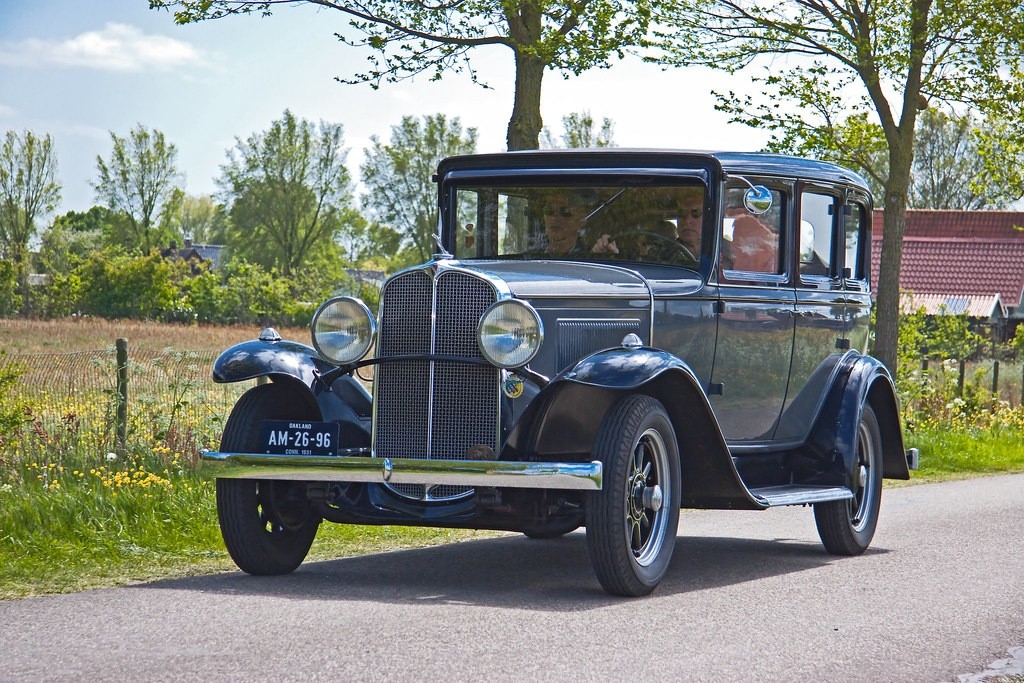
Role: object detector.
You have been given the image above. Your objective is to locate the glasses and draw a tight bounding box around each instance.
[677,207,703,219]
[543,205,581,218]
[642,238,661,246]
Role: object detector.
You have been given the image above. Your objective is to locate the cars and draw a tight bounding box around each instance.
[195,148,920,596]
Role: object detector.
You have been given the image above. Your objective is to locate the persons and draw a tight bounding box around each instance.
[591,191,734,272]
[528,191,591,253]
